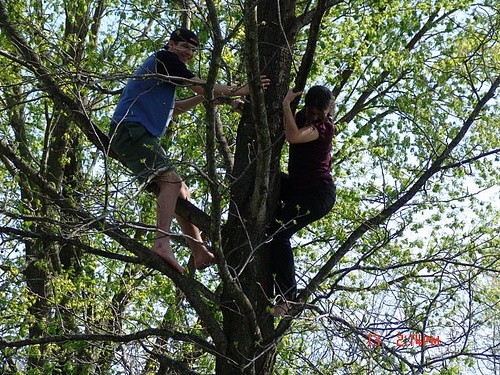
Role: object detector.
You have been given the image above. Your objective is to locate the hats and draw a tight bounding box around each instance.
[162,29,198,50]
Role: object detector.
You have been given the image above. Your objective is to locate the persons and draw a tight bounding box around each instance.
[258,85,336,316]
[109,27,271,274]
